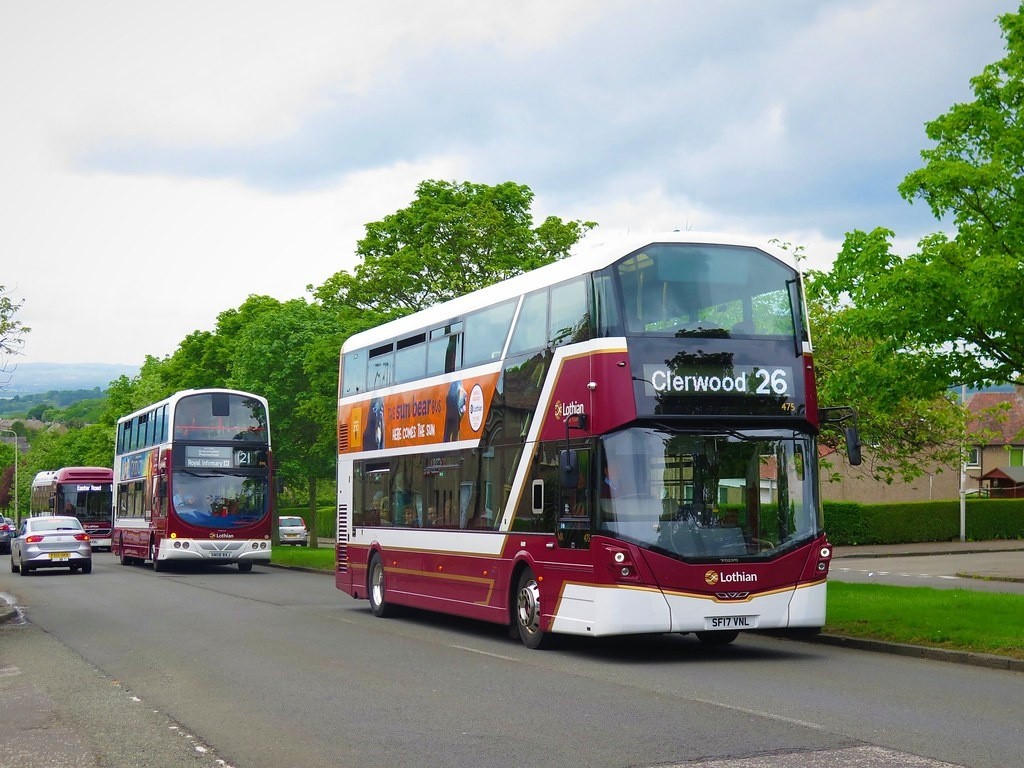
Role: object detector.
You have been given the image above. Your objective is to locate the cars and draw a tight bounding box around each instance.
[0,512,17,554]
[8,514,92,576]
[279,516,310,546]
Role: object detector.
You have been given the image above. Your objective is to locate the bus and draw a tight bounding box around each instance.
[111,389,283,571]
[335,230,865,652]
[30,466,112,554]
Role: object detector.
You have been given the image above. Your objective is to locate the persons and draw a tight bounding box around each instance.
[201,486,242,515]
[241,505,256,522]
[587,460,633,523]
[676,499,738,527]
[174,487,195,508]
[371,498,457,527]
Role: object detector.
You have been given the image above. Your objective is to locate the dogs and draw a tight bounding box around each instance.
[445,380,467,445]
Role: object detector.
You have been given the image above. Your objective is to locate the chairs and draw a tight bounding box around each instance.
[185,428,247,440]
[364,509,486,528]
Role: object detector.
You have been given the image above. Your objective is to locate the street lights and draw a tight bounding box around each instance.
[2,429,18,530]
